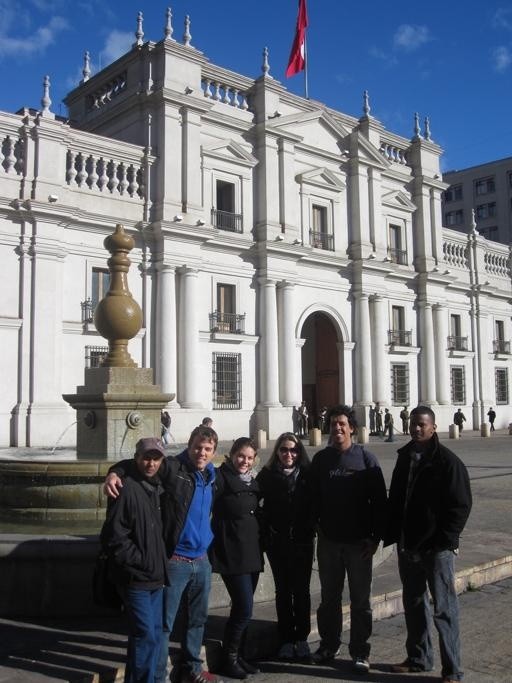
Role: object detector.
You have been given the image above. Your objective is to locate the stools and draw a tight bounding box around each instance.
[170,554,210,563]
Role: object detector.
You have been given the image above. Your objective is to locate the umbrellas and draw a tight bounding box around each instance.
[391,659,432,672]
[442,677,462,683]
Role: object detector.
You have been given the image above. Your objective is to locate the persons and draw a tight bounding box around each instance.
[374,402,382,432]
[101,437,166,682]
[383,406,471,682]
[400,405,409,434]
[199,415,213,430]
[320,406,327,431]
[383,409,394,437]
[104,423,221,683]
[208,437,274,677]
[302,407,392,672]
[453,408,466,434]
[486,406,495,430]
[257,432,318,659]
[298,400,309,437]
[162,411,171,444]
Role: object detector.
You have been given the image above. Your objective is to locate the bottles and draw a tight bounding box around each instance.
[136,437,165,456]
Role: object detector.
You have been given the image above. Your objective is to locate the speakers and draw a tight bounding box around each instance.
[220,641,262,679]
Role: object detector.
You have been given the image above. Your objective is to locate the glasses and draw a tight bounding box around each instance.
[444,268,451,274]
[383,255,391,261]
[47,193,58,204]
[368,252,376,259]
[294,238,303,244]
[433,266,440,272]
[275,234,284,241]
[196,217,206,226]
[173,213,184,223]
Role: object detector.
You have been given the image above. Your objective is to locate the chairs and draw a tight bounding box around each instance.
[311,644,341,662]
[182,670,219,683]
[295,641,311,659]
[278,642,294,658]
[351,654,370,674]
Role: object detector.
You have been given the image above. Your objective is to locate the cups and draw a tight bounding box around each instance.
[279,446,299,453]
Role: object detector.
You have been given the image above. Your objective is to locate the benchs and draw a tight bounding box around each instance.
[285,0,308,77]
